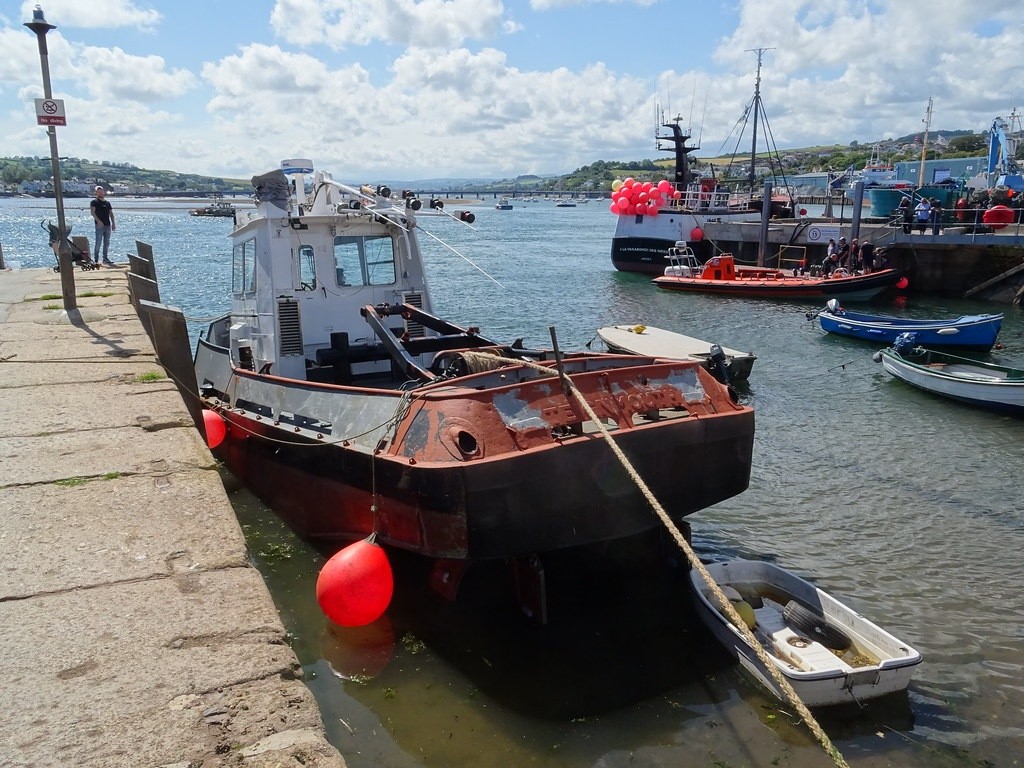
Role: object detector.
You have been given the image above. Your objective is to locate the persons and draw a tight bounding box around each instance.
[821,236,889,279]
[929,197,942,236]
[898,197,914,235]
[90,185,116,263]
[964,184,1024,235]
[914,198,931,235]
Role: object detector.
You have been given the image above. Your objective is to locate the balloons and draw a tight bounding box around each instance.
[895,296,906,307]
[897,277,908,288]
[690,228,704,240]
[202,408,227,449]
[610,178,681,216]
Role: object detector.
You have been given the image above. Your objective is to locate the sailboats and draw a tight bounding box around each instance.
[693,48,801,220]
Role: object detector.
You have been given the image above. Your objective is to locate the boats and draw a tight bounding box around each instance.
[596,324,757,379]
[806,298,1004,352]
[651,240,899,304]
[689,559,923,707]
[194,158,755,600]
[188,201,236,217]
[577,201,588,203]
[557,201,576,207]
[873,346,1024,413]
[610,192,1024,290]
[596,199,602,201]
[495,200,513,210]
[868,117,1024,219]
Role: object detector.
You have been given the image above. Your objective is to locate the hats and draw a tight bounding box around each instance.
[94,186,103,191]
[839,236,846,240]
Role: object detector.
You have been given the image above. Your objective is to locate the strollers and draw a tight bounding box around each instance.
[41,219,102,273]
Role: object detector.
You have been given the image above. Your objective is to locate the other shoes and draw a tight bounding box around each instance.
[103,259,114,263]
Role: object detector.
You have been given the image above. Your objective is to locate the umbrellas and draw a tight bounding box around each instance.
[315,538,394,627]
